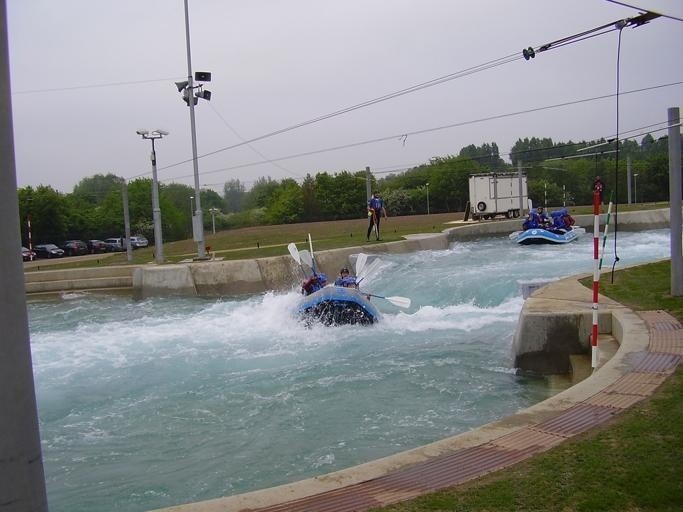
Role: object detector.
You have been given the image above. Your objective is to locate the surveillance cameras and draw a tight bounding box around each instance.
[137,131,149,134]
[157,130,169,135]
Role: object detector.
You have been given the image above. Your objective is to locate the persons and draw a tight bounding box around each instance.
[592,174,607,214]
[301,267,372,302]
[522,206,577,235]
[365,189,389,242]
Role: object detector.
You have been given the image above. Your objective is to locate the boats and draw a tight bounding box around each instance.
[515,226,582,245]
[298,285,384,328]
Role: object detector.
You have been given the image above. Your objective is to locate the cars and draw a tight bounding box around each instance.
[22,236,149,262]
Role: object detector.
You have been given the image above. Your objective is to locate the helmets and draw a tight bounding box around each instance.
[342,277,355,284]
[319,274,326,281]
[537,206,543,212]
[551,209,567,218]
[530,209,537,215]
[342,267,349,274]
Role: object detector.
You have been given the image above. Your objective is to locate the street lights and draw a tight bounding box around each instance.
[137,130,169,264]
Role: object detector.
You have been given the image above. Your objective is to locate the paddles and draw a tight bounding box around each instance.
[355,257,383,286]
[570,228,585,233]
[354,253,368,277]
[288,243,307,280]
[509,231,523,240]
[550,227,567,233]
[298,249,323,287]
[362,293,411,308]
[308,233,316,276]
